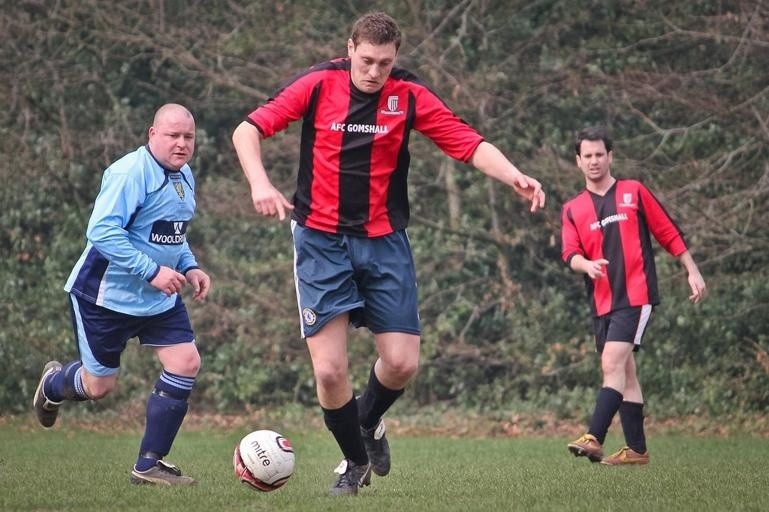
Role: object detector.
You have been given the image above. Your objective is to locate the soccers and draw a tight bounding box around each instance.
[233,429,296,492]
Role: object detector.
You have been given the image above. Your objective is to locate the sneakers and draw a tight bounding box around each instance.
[600,446,648,465]
[566,434,603,462]
[328,458,372,496]
[355,395,390,476]
[33,361,63,427]
[130,460,199,489]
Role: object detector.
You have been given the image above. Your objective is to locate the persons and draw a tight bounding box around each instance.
[560,126,705,466]
[230,11,546,499]
[33,103,212,487]
